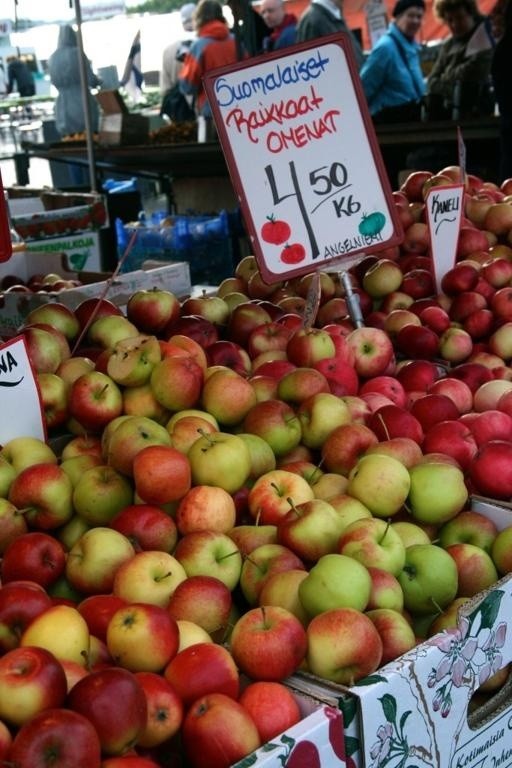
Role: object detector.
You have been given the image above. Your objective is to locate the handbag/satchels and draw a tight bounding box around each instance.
[386,31,425,125]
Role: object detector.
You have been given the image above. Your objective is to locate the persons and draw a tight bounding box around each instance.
[430,0,496,176]
[360,0,428,193]
[455,0,512,185]
[4,58,37,96]
[262,2,296,52]
[176,3,250,123]
[49,24,104,140]
[298,1,366,76]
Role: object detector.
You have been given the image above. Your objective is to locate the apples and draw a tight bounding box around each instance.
[0,286,512,768]
[220,167,512,294]
